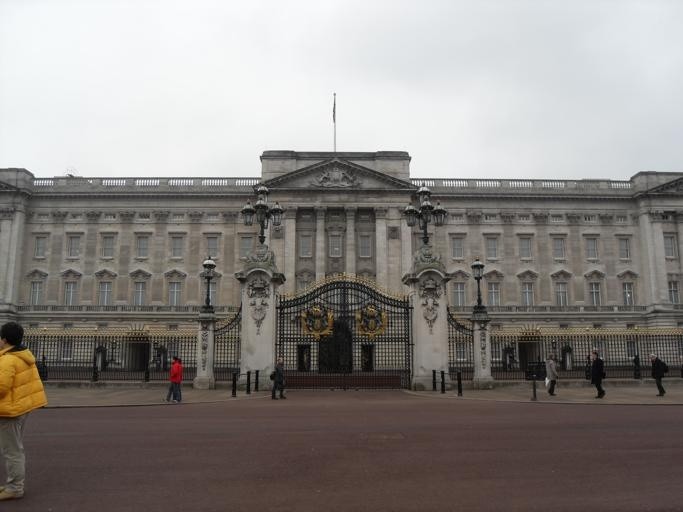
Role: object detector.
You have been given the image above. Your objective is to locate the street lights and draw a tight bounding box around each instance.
[471,256,487,313]
[199,255,221,313]
[404,182,447,263]
[240,183,284,261]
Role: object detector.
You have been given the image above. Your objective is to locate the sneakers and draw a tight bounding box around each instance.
[0,485,25,500]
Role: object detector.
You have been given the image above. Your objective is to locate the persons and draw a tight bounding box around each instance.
[0,322,48,500]
[591,352,605,398]
[546,354,558,395]
[272,359,286,400]
[161,356,182,403]
[649,354,666,396]
